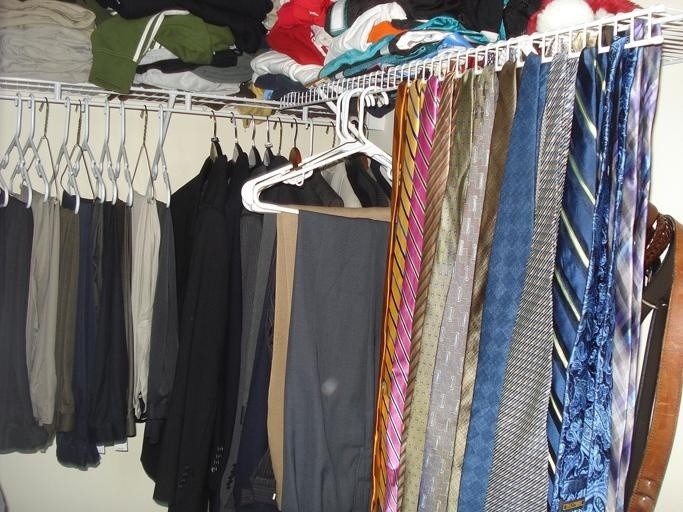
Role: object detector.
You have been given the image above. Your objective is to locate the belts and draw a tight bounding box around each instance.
[627,199,683,510]
